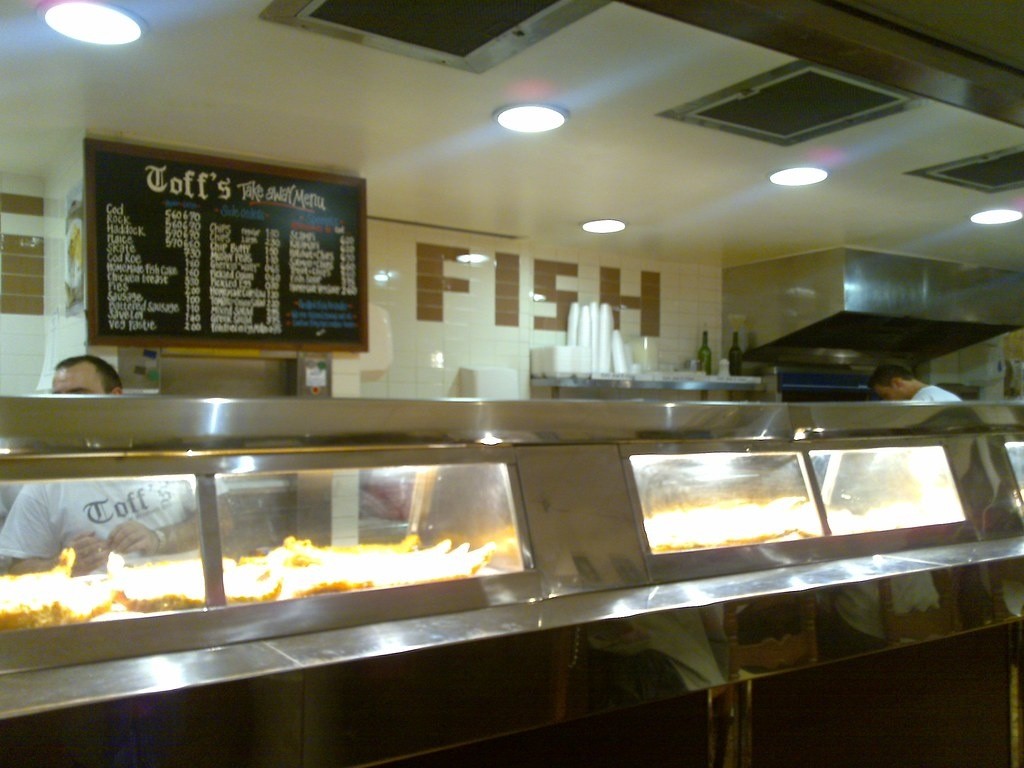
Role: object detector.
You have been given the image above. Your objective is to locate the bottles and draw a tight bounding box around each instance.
[729,332,742,375]
[717,357,729,378]
[698,331,711,375]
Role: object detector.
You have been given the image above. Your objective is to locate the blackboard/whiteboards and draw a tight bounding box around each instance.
[84,134,371,352]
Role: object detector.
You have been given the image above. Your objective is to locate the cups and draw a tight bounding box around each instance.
[567,300,636,375]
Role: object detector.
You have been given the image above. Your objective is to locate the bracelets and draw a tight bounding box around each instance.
[156,531,166,553]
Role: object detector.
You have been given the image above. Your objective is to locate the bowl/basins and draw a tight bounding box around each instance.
[531,345,591,379]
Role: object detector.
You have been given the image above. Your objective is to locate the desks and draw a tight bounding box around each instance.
[529,373,766,404]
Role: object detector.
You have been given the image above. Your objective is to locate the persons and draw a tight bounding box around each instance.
[871,364,973,482]
[0,356,236,579]
